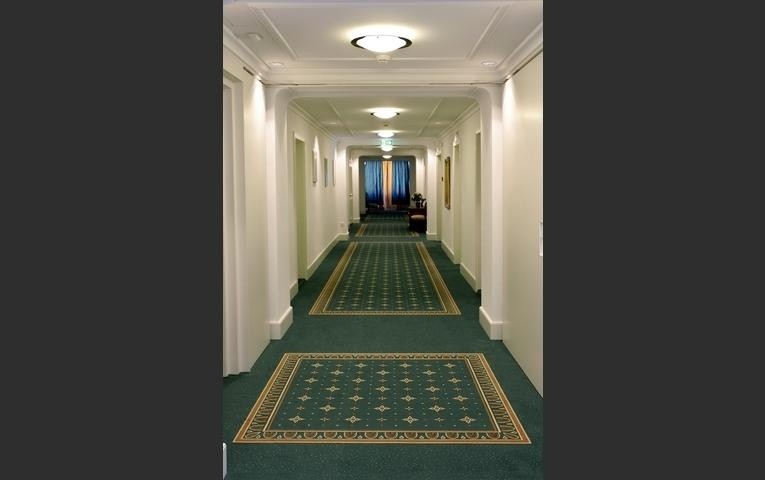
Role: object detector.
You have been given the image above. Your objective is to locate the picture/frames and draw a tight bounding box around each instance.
[443,156,450,209]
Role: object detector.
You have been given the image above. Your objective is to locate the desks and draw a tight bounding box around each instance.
[407,206,427,230]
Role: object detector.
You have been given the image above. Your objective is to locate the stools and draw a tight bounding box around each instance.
[411,215,425,232]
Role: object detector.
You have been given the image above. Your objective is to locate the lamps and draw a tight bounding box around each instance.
[371,112,400,120]
[351,36,412,52]
[377,133,394,159]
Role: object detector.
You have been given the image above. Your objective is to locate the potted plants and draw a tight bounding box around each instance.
[412,192,422,208]
